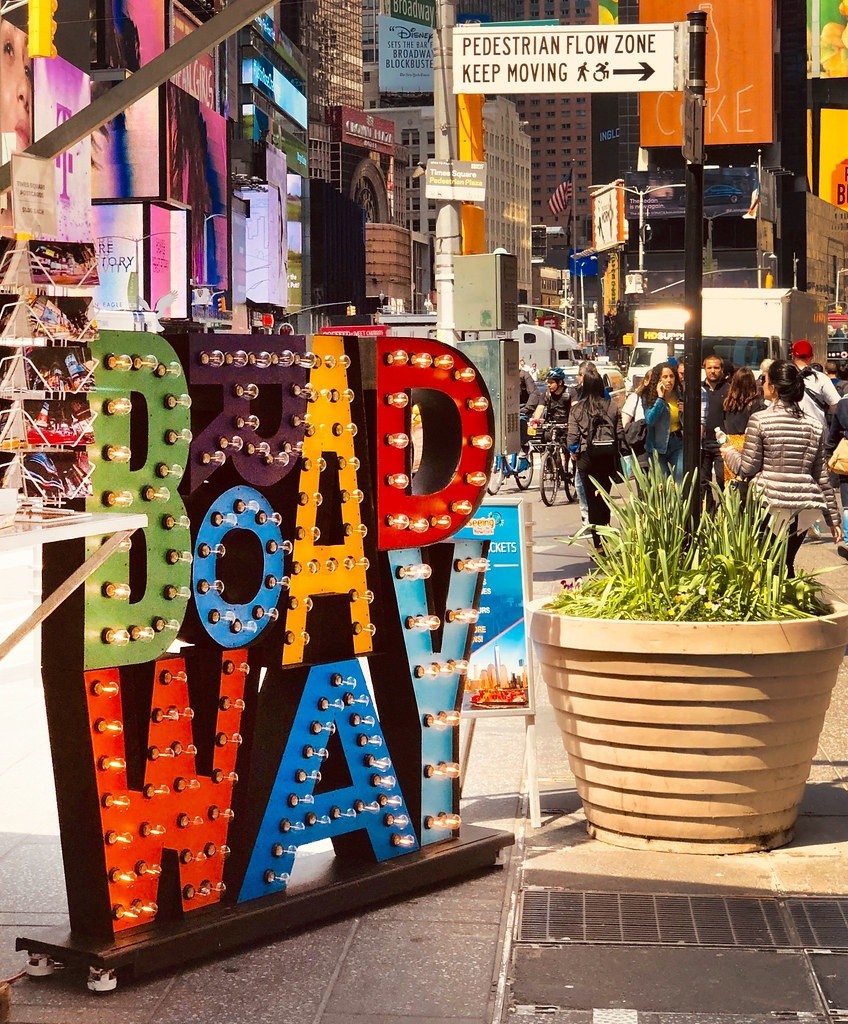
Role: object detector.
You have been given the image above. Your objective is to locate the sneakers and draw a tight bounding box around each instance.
[590,547,605,560]
[513,459,530,473]
[808,521,821,540]
[837,543,848,563]
[569,528,593,539]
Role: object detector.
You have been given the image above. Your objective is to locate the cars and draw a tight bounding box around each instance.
[679,185,743,207]
[530,360,627,451]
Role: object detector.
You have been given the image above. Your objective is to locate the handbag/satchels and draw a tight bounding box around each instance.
[827,437,848,475]
[620,418,647,457]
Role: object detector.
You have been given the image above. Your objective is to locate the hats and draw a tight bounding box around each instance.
[792,340,814,362]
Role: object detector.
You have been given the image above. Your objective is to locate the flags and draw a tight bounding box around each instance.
[549,167,573,214]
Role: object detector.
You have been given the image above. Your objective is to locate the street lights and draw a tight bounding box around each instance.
[96,231,177,313]
[757,252,777,288]
[203,213,226,286]
[588,182,685,270]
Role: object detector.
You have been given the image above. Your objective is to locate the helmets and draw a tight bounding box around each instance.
[546,368,566,379]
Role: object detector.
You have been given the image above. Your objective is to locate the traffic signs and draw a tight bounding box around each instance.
[682,87,694,161]
[452,26,676,93]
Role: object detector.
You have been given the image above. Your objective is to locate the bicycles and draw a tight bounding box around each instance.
[532,419,579,507]
[486,411,534,495]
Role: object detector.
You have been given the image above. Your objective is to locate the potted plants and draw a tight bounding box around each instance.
[526,444,848,854]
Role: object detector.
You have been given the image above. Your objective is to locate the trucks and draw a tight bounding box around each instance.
[626,309,684,395]
[378,314,585,370]
[702,287,829,380]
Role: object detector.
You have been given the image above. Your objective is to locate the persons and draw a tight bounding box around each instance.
[0,18,36,156]
[517,339,848,560]
[719,359,842,582]
